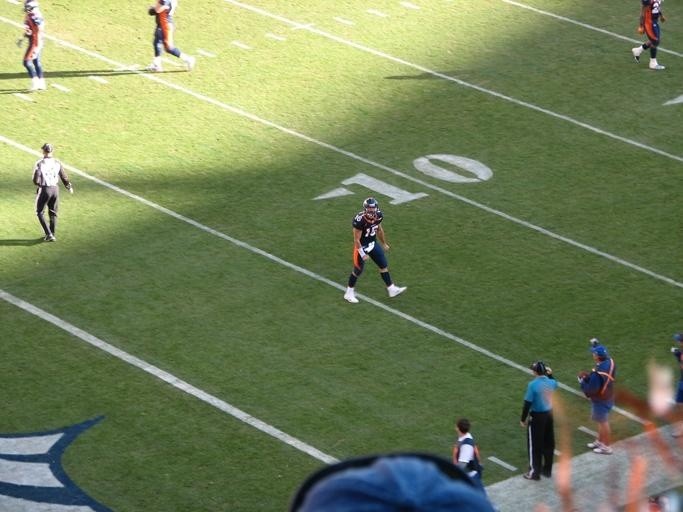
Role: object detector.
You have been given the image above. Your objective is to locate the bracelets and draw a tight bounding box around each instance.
[357,248,366,258]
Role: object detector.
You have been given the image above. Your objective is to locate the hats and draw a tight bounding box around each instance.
[529,362,547,375]
[40,143,52,152]
[591,346,607,356]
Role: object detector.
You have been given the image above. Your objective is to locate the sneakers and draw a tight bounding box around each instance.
[343,287,360,304]
[188,57,195,69]
[386,284,407,298]
[593,443,612,454]
[587,440,601,448]
[38,79,45,89]
[145,63,162,70]
[29,78,40,91]
[632,46,644,63]
[523,472,541,481]
[43,233,56,242]
[648,58,664,69]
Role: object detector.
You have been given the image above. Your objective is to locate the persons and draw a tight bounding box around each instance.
[31,141,74,244]
[341,195,408,305]
[147,1,194,75]
[15,0,47,93]
[576,333,617,455]
[449,417,483,489]
[518,360,558,481]
[667,324,682,435]
[629,1,666,72]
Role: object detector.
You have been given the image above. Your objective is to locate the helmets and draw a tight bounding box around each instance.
[362,197,379,219]
